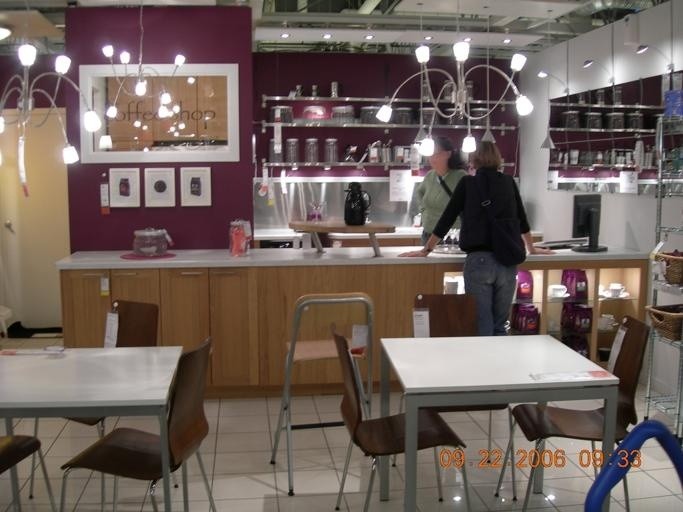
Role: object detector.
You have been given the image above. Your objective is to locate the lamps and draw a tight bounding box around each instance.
[537,68,569,94]
[102,0,185,118]
[0,0,102,166]
[413,2,428,143]
[375,0,535,155]
[479,13,497,143]
[584,58,614,84]
[636,44,674,71]
[540,9,556,148]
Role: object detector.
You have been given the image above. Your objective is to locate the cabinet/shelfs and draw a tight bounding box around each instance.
[59,258,651,402]
[105,76,227,151]
[260,93,518,168]
[549,101,670,170]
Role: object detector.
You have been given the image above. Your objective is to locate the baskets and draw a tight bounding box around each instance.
[643,252,683,340]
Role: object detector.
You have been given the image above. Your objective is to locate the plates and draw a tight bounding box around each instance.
[546,294,569,301]
[604,293,630,299]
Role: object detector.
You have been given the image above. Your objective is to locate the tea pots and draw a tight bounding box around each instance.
[343,182,371,226]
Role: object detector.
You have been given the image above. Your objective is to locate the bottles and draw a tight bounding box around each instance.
[273,105,487,126]
[561,87,643,130]
[270,138,338,164]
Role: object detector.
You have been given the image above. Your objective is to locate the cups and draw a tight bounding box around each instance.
[619,172,639,194]
[530,232,543,242]
[600,314,615,330]
[547,171,559,189]
[369,148,379,162]
[551,285,567,294]
[609,285,624,294]
[636,140,653,166]
[446,281,458,293]
[570,150,579,164]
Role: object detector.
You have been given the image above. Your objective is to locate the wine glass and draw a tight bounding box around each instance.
[442,228,460,250]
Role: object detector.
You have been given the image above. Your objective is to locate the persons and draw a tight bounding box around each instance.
[398,137,557,336]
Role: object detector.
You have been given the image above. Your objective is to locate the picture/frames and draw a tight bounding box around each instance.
[180,166,212,207]
[143,167,175,208]
[108,167,141,208]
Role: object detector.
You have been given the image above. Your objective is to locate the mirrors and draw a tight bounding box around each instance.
[549,70,683,172]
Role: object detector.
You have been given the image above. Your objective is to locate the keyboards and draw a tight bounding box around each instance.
[531,236,588,249]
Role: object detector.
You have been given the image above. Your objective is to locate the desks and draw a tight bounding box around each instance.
[377,333,621,512]
[0,345,184,512]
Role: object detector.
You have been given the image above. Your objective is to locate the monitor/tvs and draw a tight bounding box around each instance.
[570,194,609,253]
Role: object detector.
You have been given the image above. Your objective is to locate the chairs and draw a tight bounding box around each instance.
[329,322,472,512]
[270,290,381,497]
[59,336,218,512]
[0,436,59,512]
[393,293,518,502]
[493,314,651,512]
[27,298,159,512]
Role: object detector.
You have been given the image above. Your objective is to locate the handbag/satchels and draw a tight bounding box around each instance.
[494,239,527,267]
